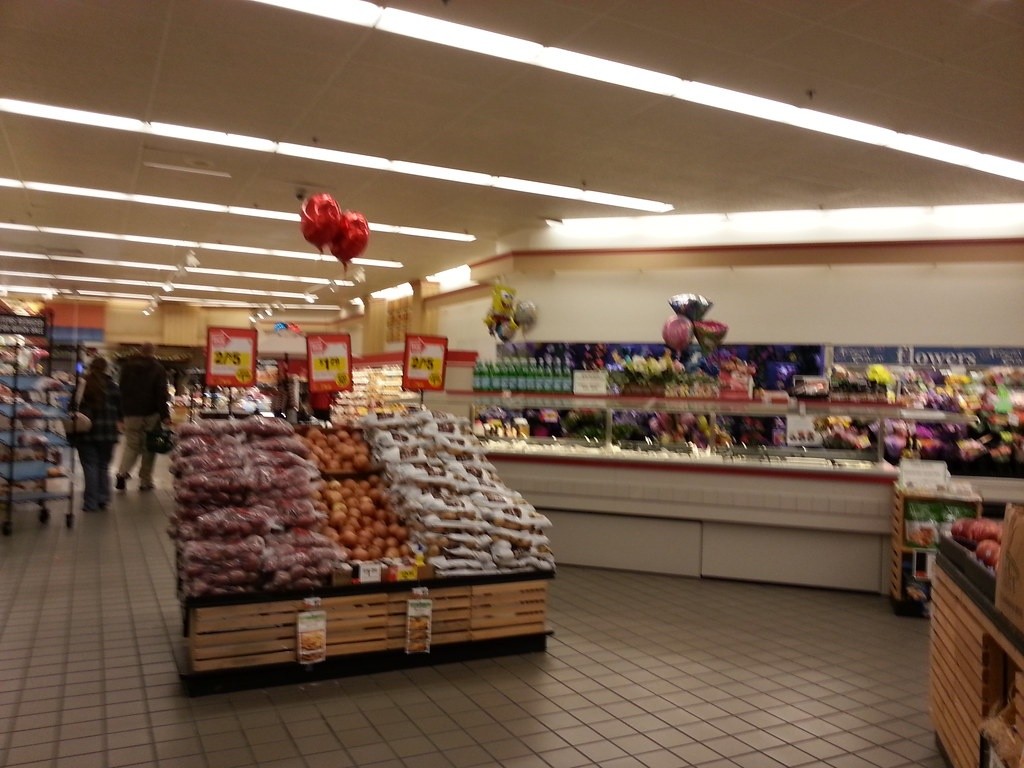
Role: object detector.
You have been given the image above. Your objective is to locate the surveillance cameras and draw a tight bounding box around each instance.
[296,192,305,200]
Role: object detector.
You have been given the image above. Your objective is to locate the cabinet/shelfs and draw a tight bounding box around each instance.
[330,350,479,424]
[0,342,80,536]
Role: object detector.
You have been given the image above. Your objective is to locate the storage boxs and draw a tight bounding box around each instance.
[887,537,933,618]
[890,482,984,550]
[994,503,1024,632]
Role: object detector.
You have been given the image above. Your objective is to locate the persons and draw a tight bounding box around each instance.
[116,343,171,490]
[77,357,120,512]
[308,390,335,421]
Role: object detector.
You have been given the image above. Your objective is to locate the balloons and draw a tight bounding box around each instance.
[328,211,370,268]
[663,294,728,358]
[302,193,341,253]
[485,285,538,342]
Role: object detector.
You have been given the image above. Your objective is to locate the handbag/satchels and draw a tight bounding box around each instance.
[64,378,92,432]
[148,422,179,454]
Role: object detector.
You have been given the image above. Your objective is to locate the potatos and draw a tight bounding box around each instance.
[951,517,1003,570]
[292,426,411,562]
[166,417,351,594]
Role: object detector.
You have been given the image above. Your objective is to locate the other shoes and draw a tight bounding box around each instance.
[140,485,155,491]
[116,477,126,489]
